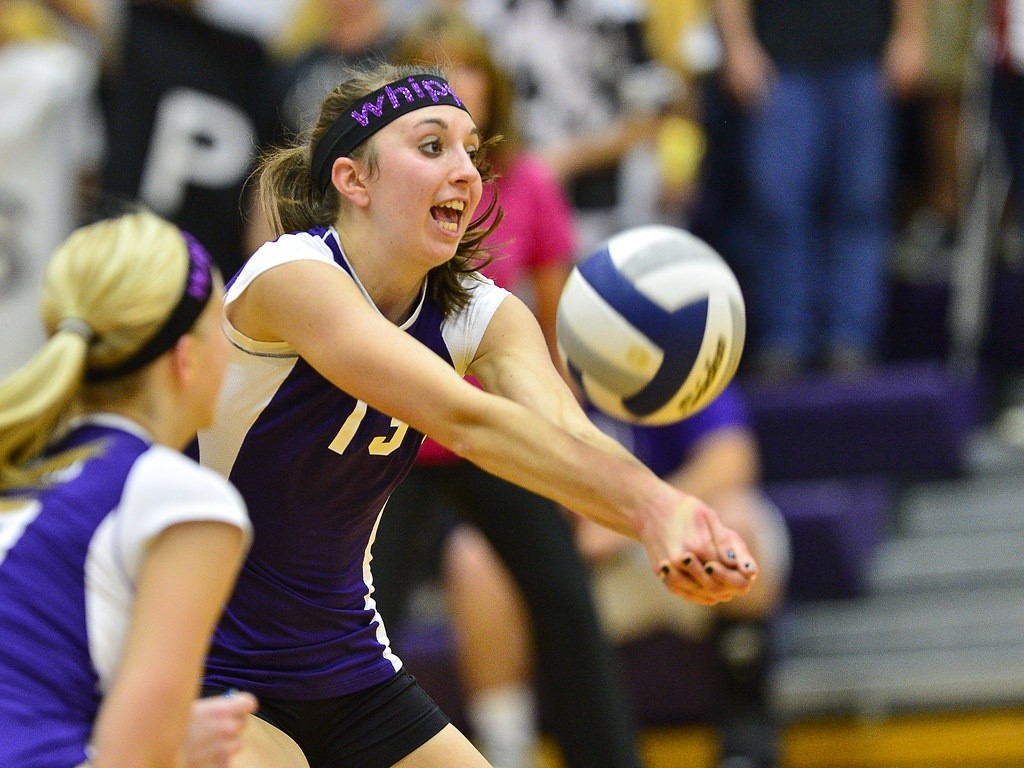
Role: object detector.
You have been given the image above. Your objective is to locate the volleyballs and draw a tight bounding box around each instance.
[554,219,748,428]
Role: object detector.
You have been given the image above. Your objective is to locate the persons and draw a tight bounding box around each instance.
[1,0,789,768]
[719,0,933,377]
[950,0,1024,476]
[182,62,759,768]
[1,209,260,768]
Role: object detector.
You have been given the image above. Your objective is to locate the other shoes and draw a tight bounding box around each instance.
[714,734,780,768]
